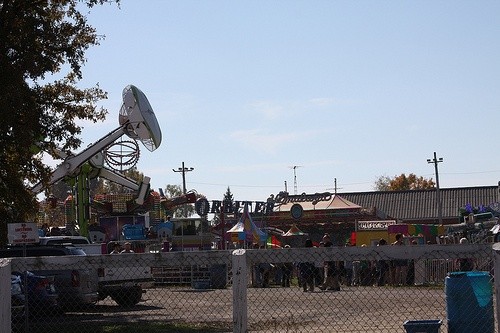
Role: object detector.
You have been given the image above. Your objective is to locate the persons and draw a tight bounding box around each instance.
[120,242,134,253]
[110,244,122,254]
[455,237,474,272]
[249,232,418,292]
[37,223,50,238]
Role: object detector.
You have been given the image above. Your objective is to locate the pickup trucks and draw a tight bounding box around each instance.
[39,236,156,306]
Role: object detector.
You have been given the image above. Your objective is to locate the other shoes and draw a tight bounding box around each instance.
[327,287,333,290]
[307,288,314,291]
[275,282,290,287]
[318,285,324,290]
[303,290,307,292]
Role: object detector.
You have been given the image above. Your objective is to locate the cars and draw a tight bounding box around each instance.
[0,244,99,317]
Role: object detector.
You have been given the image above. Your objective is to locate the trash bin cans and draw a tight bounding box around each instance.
[404,319,443,333]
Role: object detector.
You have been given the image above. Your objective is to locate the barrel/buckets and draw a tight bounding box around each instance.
[444,270,494,333]
